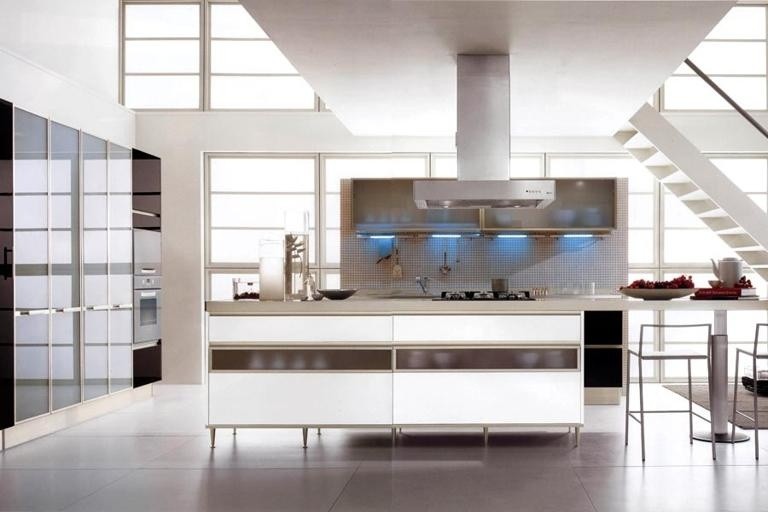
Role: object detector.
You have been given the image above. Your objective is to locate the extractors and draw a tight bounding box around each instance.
[412,56,557,211]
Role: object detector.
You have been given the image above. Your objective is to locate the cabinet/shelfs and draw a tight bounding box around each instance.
[0,96,164,456]
[204,296,631,451]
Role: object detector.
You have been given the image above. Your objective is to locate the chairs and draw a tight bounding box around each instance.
[729,320,768,462]
[617,320,717,467]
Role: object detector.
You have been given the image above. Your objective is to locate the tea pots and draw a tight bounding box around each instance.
[710,254,748,287]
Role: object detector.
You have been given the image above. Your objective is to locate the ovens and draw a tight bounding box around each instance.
[131,227,164,343]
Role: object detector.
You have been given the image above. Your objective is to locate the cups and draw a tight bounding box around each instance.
[232,210,309,304]
[492,278,511,296]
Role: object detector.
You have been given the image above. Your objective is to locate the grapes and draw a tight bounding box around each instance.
[627,275,694,289]
[735,275,752,288]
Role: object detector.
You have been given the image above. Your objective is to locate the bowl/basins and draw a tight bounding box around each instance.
[708,278,720,288]
[316,287,357,301]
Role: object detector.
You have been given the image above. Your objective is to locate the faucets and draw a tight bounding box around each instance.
[415,276,429,294]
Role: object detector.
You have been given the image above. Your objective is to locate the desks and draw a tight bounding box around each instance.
[583,294,768,445]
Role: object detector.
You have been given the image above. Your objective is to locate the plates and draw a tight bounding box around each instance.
[622,286,699,301]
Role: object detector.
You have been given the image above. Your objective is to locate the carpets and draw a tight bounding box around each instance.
[663,381,766,431]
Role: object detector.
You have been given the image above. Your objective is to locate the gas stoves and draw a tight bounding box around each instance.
[432,289,538,301]
